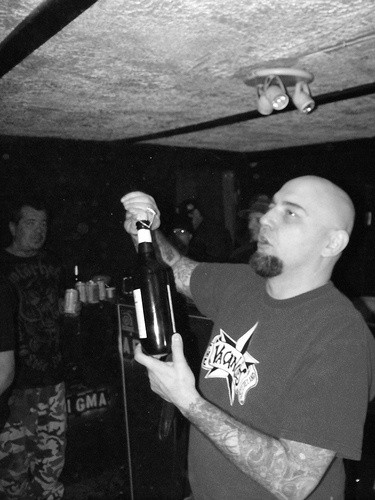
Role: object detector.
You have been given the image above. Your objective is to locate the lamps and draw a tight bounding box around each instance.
[245,66,316,120]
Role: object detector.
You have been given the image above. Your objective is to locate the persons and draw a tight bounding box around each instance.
[0,188,112,500]
[118,175,375,500]
[164,194,271,316]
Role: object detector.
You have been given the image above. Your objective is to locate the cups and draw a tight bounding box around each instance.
[107,288,115,298]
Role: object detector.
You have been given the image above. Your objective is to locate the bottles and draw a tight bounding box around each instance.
[72,263,83,286]
[131,214,177,357]
